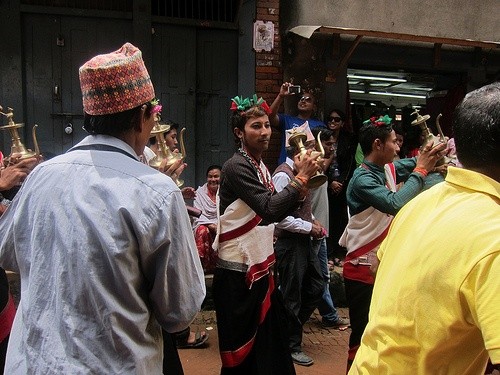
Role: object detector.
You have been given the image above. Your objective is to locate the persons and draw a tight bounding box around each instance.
[211,95,323,375]
[0,42,208,375]
[347,81,500,375]
[339,117,451,375]
[151,121,211,349]
[270,79,351,330]
[271,120,328,366]
[0,149,44,375]
[355,128,463,193]
[193,164,220,269]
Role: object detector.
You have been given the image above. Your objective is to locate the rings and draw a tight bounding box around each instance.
[306,153,310,156]
[423,147,429,152]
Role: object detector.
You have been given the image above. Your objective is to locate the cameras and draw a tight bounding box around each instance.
[288,85,301,93]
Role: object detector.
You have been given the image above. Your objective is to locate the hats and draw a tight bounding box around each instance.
[285,121,315,148]
[79,42,155,115]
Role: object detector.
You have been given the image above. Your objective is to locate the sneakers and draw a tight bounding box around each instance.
[320,315,351,330]
[292,351,313,365]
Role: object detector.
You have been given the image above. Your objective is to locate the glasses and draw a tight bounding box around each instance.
[301,96,312,102]
[329,116,341,122]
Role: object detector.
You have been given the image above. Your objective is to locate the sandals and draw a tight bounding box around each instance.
[177,331,209,348]
